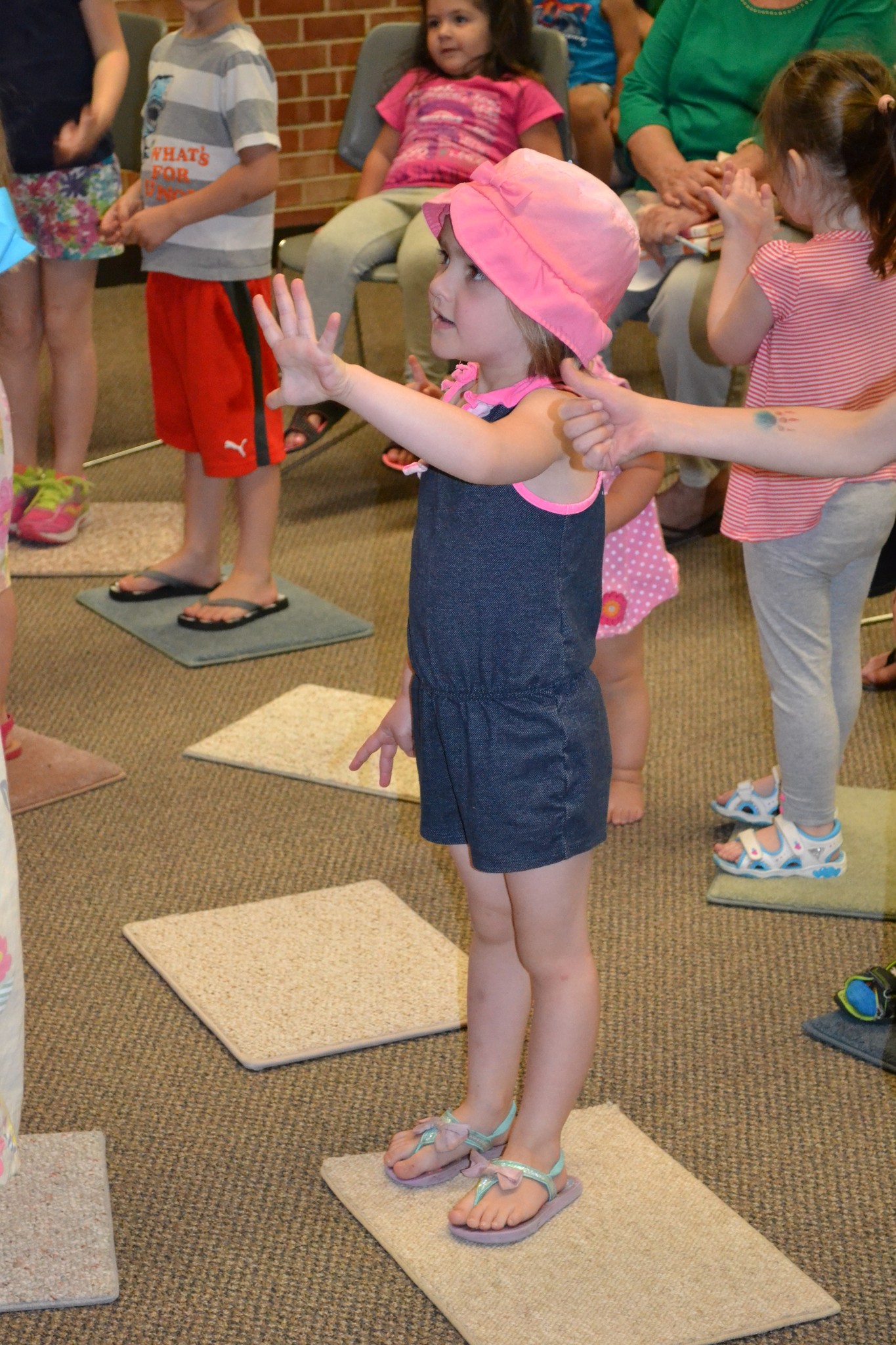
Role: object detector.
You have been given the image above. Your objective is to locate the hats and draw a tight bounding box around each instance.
[423,148,641,370]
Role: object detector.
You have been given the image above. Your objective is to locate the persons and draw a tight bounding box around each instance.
[280,0,567,475]
[698,45,896,881]
[576,1,896,553]
[0,0,131,546]
[529,0,644,186]
[402,347,683,825]
[95,0,289,634]
[251,146,645,1249]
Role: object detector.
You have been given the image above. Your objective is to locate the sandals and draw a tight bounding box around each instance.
[713,814,847,879]
[282,400,349,453]
[834,960,896,1024]
[710,764,781,826]
[382,442,419,473]
[384,1100,516,1188]
[448,1147,584,1243]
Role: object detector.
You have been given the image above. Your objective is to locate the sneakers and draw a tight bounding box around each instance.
[8,464,42,537]
[17,473,91,546]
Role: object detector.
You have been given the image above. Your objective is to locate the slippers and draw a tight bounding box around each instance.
[0,713,22,759]
[177,594,289,631]
[109,567,221,603]
[654,507,724,550]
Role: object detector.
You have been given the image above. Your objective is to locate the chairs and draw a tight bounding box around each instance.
[272,19,572,371]
[71,6,171,473]
